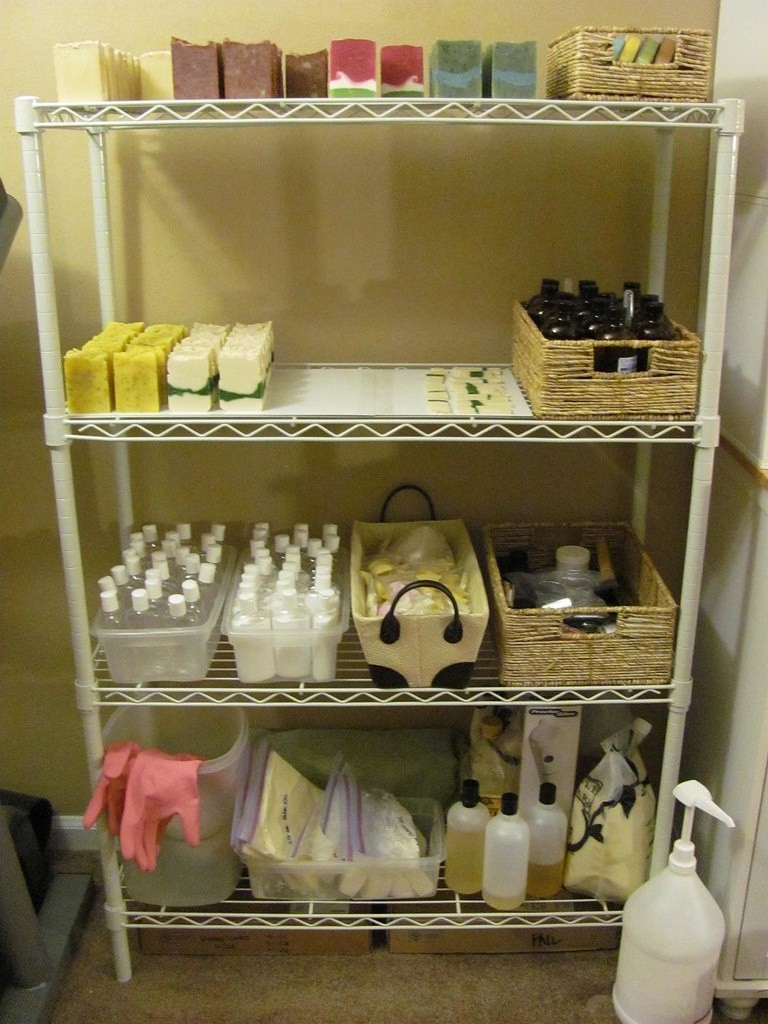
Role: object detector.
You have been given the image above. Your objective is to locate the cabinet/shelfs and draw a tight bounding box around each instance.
[11,92,745,983]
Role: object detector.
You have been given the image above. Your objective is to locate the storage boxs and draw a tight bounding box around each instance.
[386,865,619,954]
[239,796,446,903]
[222,546,349,684]
[139,883,377,958]
[517,705,584,819]
[87,544,238,684]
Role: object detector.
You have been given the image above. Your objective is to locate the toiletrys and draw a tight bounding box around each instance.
[231,523,344,680]
[100,522,226,674]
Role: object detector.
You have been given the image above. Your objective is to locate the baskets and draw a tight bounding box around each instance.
[484,521,676,684]
[512,300,701,421]
[546,26,715,104]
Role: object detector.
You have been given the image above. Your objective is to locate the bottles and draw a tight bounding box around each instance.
[459,717,519,801]
[525,781,568,899]
[96,520,345,682]
[444,778,489,895]
[482,791,531,912]
[526,277,680,374]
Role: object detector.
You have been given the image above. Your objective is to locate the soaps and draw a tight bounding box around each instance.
[285,38,427,102]
[601,32,677,66]
[433,38,539,100]
[53,38,282,98]
[62,322,275,416]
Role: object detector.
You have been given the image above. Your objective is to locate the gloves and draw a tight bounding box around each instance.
[120,748,211,872]
[83,739,144,834]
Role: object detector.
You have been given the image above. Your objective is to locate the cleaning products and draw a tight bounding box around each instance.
[614,777,734,1024]
[444,779,569,913]
